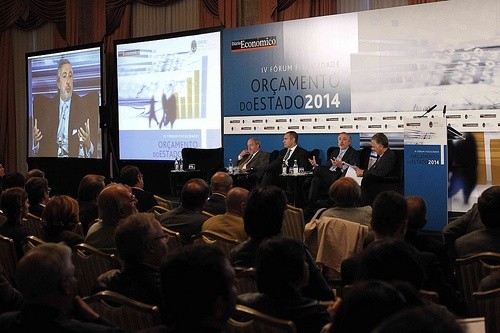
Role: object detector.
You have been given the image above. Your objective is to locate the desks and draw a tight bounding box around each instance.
[169,171,313,213]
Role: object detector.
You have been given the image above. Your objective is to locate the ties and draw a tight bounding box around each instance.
[239,154,253,169]
[58,103,68,157]
[282,150,292,167]
[331,152,343,170]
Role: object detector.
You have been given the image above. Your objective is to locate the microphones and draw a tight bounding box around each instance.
[443,105,446,118]
[422,105,437,116]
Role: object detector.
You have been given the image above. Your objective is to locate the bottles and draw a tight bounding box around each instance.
[282,160,287,174]
[293,159,298,174]
[175,158,183,170]
[229,159,233,173]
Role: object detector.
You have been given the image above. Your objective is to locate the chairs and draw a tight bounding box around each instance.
[0,148,500,333]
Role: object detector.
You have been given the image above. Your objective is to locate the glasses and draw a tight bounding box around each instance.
[147,233,170,244]
[44,187,51,194]
[122,195,136,205]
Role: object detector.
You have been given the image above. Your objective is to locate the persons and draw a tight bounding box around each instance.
[304,132,361,225]
[259,132,307,214]
[235,138,266,187]
[351,133,402,208]
[446,132,478,204]
[0,159,500,333]
[29,58,102,159]
[144,84,178,130]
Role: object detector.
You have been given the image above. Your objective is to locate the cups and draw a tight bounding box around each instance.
[289,168,304,174]
